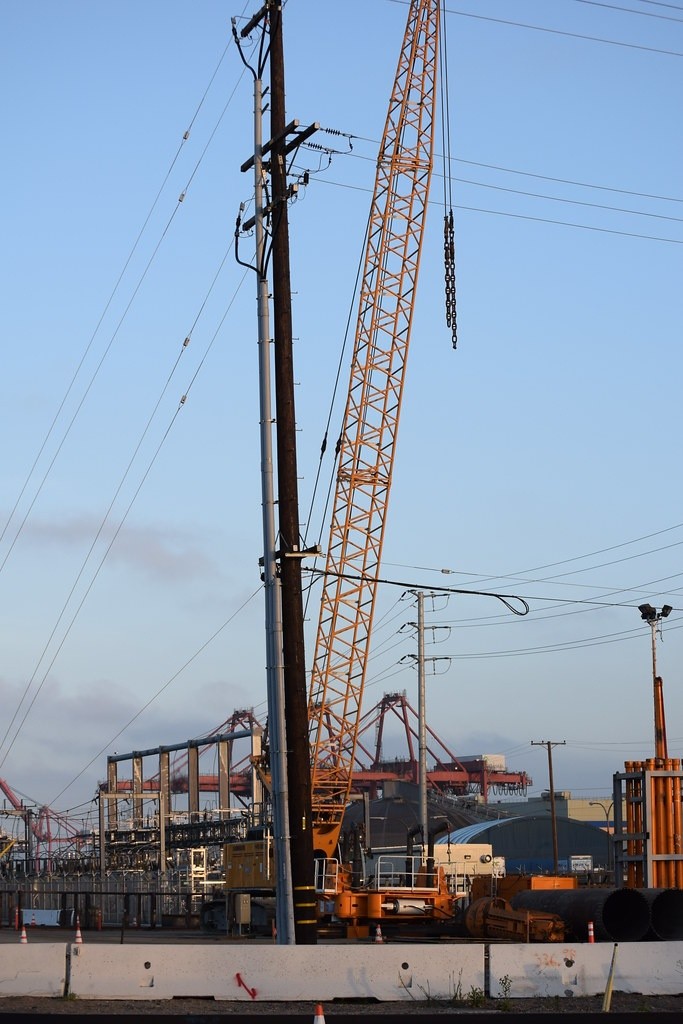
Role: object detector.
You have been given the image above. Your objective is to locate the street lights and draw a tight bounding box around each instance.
[589,797,626,881]
[637,603,674,759]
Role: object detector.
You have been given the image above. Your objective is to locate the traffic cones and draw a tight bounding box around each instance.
[76,914,81,930]
[132,914,137,927]
[374,924,384,944]
[313,1004,326,1024]
[74,927,83,944]
[19,927,27,944]
[31,913,36,926]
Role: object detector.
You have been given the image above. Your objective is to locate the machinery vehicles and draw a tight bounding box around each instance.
[223,0,457,941]
